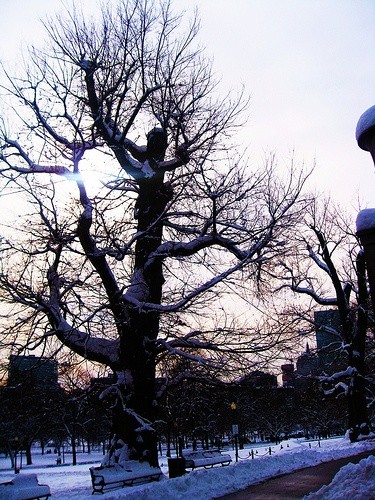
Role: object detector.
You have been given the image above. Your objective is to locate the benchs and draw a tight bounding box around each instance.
[89,460,163,495]
[183,449,232,472]
[0,474,52,500]
